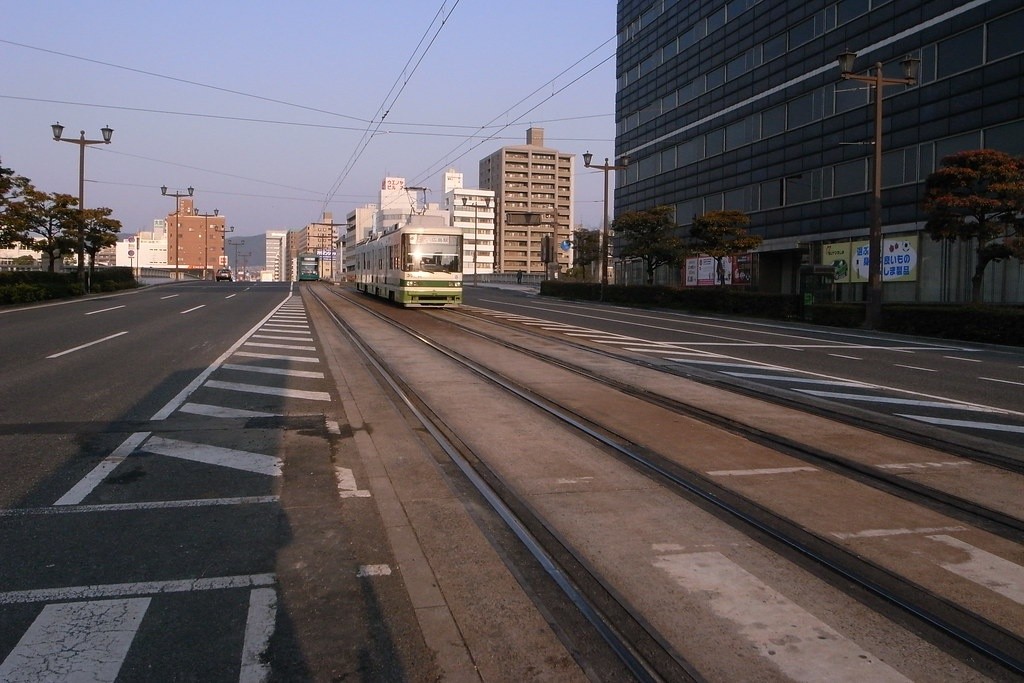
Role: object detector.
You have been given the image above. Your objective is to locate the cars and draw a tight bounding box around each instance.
[216,268,233,282]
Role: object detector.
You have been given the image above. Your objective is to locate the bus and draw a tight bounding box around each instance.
[353,185,465,310]
[296,252,320,282]
[343,259,356,285]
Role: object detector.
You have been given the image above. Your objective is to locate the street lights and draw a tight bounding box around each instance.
[227,239,245,281]
[49,120,114,294]
[237,251,252,281]
[581,149,632,304]
[194,207,220,280]
[214,225,235,267]
[835,46,923,329]
[159,184,196,281]
[462,197,492,286]
